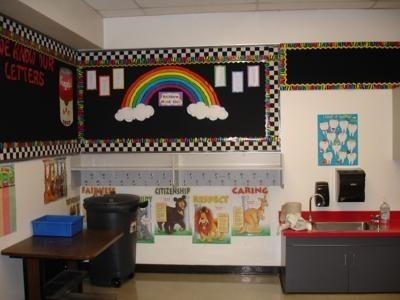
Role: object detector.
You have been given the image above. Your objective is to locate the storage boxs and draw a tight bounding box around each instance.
[32,215,84,238]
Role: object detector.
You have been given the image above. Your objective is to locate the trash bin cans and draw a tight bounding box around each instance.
[84,194,140,286]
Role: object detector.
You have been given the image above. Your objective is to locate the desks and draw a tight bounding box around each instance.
[1,229,125,300]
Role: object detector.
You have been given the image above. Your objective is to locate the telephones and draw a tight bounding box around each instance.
[280,41,400,90]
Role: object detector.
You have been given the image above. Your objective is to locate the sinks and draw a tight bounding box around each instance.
[310,222,382,231]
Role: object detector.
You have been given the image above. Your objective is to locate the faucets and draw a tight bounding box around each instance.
[308,194,326,221]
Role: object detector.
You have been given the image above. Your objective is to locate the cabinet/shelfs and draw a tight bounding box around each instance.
[280,236,400,293]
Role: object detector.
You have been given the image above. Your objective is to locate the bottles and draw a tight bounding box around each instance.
[380,200,390,220]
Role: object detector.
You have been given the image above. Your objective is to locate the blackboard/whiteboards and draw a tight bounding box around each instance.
[1,13,280,163]
